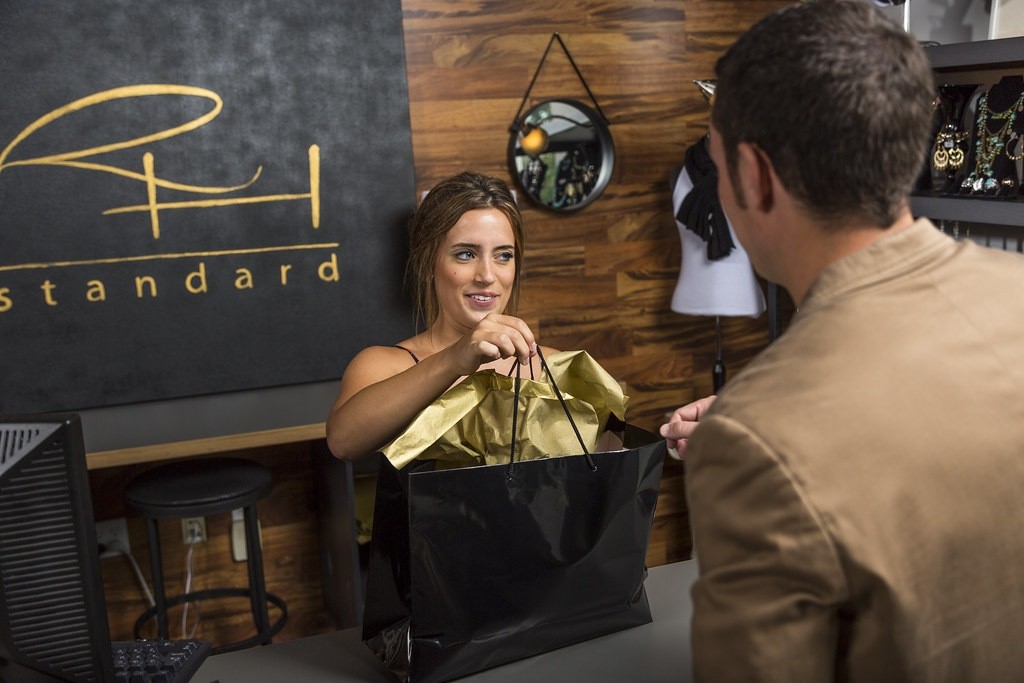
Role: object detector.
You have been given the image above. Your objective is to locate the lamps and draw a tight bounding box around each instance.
[520,112,597,155]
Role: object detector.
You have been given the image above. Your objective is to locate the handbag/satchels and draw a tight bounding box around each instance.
[361,343,668,683]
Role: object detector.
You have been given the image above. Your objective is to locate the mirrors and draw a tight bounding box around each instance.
[509,103,618,212]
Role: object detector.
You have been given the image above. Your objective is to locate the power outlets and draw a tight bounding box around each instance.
[180,516,208,546]
[96,516,131,561]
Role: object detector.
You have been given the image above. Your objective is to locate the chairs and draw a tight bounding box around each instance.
[126,457,289,654]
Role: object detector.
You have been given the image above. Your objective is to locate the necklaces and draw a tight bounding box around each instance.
[933,90,1024,193]
[523,151,595,205]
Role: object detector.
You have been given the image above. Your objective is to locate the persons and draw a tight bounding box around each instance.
[658,0,1024,683]
[326,171,564,463]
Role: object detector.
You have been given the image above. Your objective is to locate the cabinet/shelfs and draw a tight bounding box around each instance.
[910,37,1024,225]
